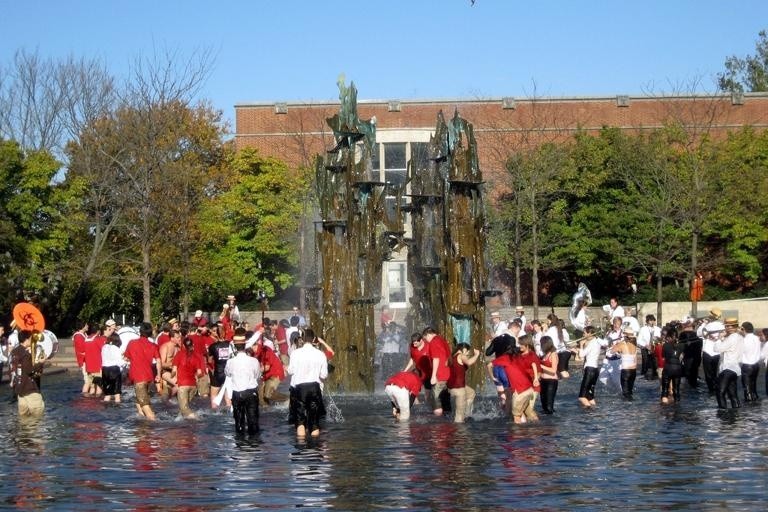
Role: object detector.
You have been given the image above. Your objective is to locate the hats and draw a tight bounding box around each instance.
[105,319,116,326]
[709,308,721,320]
[722,318,739,325]
[168,318,179,325]
[226,295,236,300]
[515,306,525,311]
[195,310,203,318]
[488,312,502,318]
[232,335,247,344]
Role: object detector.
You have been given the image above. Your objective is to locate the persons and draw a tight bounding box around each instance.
[537,337,559,414]
[256,318,270,332]
[207,326,235,409]
[757,329,768,399]
[310,335,336,362]
[123,323,163,418]
[555,319,572,374]
[531,322,544,358]
[225,319,239,343]
[208,323,219,343]
[183,323,210,398]
[284,316,302,354]
[697,306,725,397]
[85,324,105,396]
[713,316,745,410]
[101,333,127,402]
[268,321,290,377]
[659,329,682,405]
[252,326,277,356]
[0,326,8,384]
[287,331,330,436]
[736,323,762,403]
[168,319,180,332]
[421,328,452,418]
[578,325,602,408]
[157,322,174,347]
[97,325,110,345]
[491,321,519,350]
[605,315,624,360]
[678,315,703,386]
[512,306,527,337]
[608,329,638,399]
[192,310,206,327]
[636,315,663,376]
[73,319,92,394]
[620,310,640,333]
[240,322,251,331]
[569,291,586,339]
[544,314,569,378]
[444,343,481,422]
[602,297,623,324]
[169,338,202,416]
[379,303,395,332]
[401,333,434,408]
[105,320,117,333]
[221,306,236,330]
[222,295,243,327]
[488,342,540,424]
[512,335,541,422]
[224,336,262,434]
[160,329,181,395]
[9,330,45,417]
[385,367,422,421]
